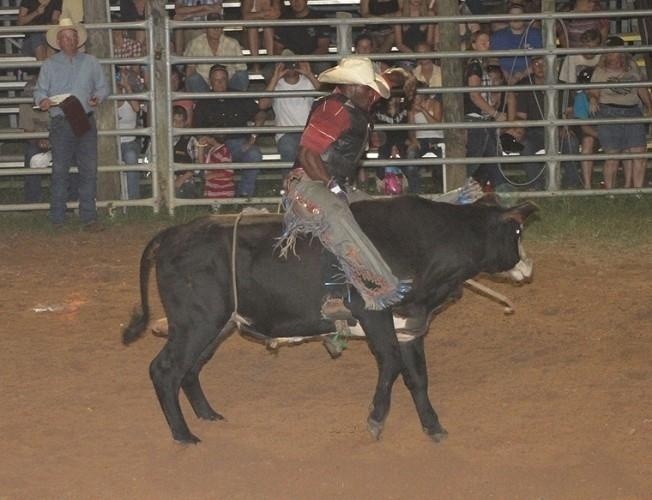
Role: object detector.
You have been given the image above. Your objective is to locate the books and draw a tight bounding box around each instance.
[32,93,92,137]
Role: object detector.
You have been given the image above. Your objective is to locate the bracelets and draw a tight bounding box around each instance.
[329,184,342,193]
[325,175,337,190]
[250,133,257,138]
[196,141,209,148]
[492,110,497,118]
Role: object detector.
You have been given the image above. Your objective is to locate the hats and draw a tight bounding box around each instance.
[500,134,524,154]
[606,36,625,47]
[46,17,89,50]
[317,55,391,100]
[210,64,227,72]
[206,12,221,21]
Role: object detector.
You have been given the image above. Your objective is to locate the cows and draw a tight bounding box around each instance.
[117,185,539,450]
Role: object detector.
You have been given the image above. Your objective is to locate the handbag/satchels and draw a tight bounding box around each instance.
[384,167,403,195]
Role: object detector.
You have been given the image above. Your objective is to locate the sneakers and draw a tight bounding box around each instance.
[321,298,355,324]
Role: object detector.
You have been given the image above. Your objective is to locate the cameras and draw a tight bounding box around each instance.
[283,62,299,69]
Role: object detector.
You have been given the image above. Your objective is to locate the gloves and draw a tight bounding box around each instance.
[327,176,349,203]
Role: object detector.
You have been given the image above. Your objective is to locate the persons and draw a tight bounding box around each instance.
[405,79,444,193]
[489,1,542,32]
[463,30,520,194]
[193,121,236,214]
[172,1,225,56]
[120,0,149,90]
[489,6,543,121]
[17,80,79,212]
[33,16,111,234]
[169,69,195,129]
[587,36,652,200]
[360,1,401,53]
[412,42,443,90]
[352,36,388,73]
[16,0,63,62]
[172,105,201,201]
[183,13,250,94]
[558,29,604,191]
[272,0,334,89]
[257,49,321,197]
[512,54,583,192]
[192,63,266,212]
[371,69,414,193]
[110,17,148,161]
[112,65,143,206]
[284,56,417,312]
[394,1,436,69]
[429,2,472,55]
[241,0,282,76]
[555,0,610,51]
